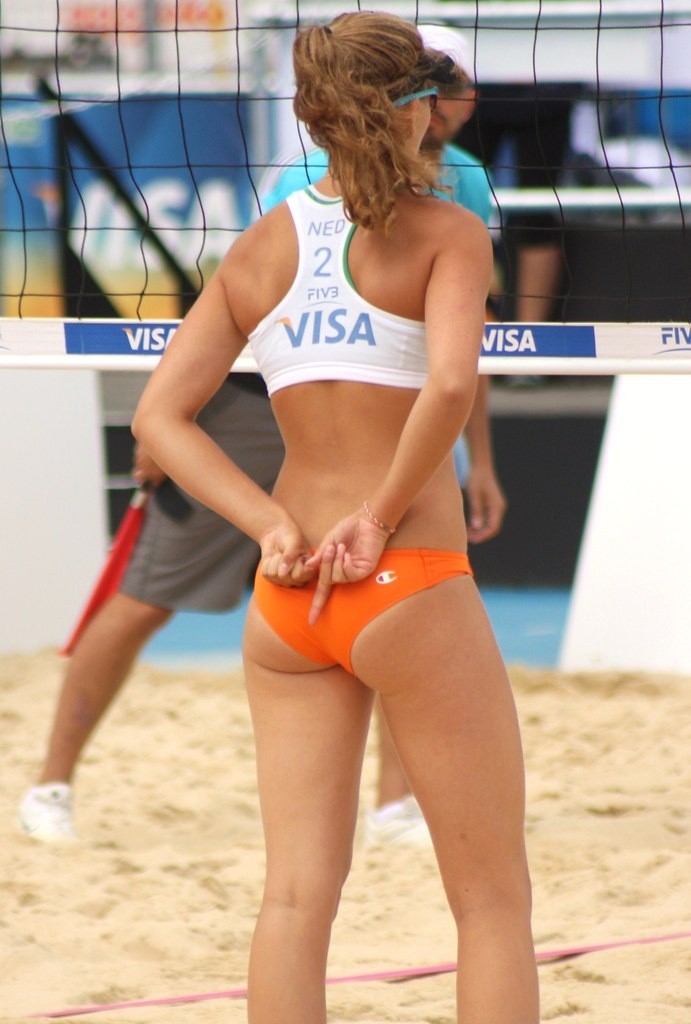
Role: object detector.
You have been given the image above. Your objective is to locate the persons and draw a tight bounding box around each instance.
[21,11,561,1024]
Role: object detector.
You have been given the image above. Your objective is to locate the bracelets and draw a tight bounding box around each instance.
[365,502,395,533]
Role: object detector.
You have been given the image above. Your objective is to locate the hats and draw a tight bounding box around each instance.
[413,21,474,81]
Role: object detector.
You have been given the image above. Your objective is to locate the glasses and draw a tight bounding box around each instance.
[393,86,438,112]
[423,71,471,95]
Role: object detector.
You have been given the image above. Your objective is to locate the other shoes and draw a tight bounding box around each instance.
[20,783,80,846]
[364,793,433,846]
